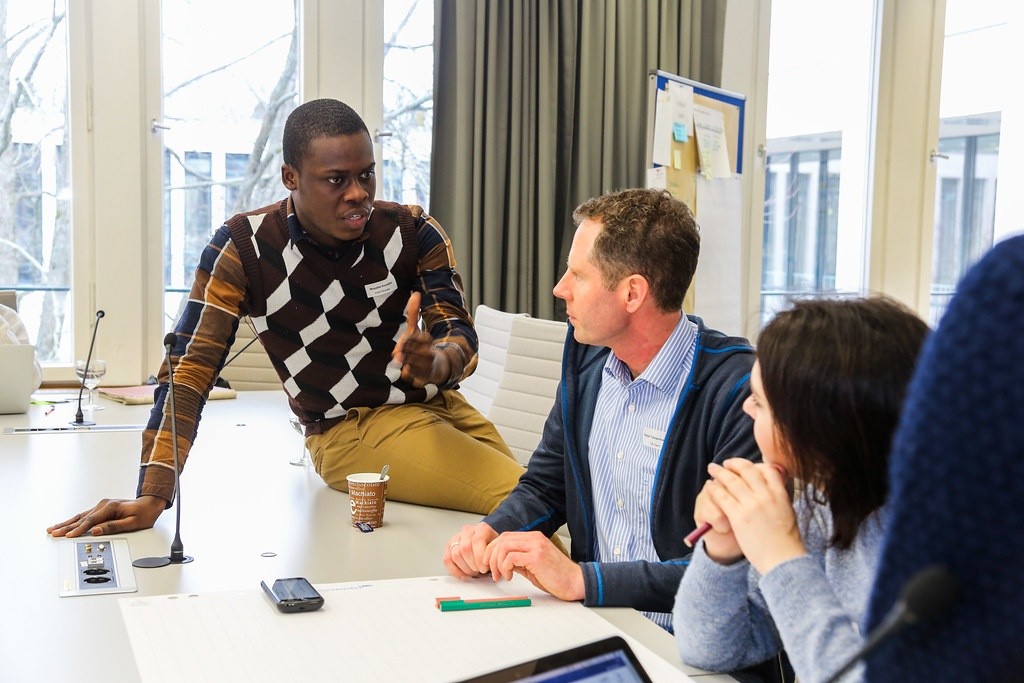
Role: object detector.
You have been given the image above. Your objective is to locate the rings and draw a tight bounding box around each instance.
[450,542,459,550]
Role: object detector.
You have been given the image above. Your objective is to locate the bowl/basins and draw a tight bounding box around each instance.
[0,344,34,414]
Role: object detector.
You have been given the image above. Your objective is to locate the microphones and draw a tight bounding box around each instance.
[162,331,184,561]
[76,310,105,423]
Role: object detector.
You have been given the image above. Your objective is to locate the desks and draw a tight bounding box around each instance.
[0,385,745,683]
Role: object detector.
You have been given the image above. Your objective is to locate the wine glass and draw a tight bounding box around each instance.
[76,360,107,411]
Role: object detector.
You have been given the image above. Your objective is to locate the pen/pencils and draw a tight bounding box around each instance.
[684,523,712,549]
[45,405,54,415]
[435,596,528,609]
[440,598,531,612]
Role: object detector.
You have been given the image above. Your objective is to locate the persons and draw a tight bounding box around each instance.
[670,231,1024,683]
[45,98,570,558]
[443,189,761,634]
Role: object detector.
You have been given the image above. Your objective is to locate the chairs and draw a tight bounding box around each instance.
[212,315,288,392]
[462,303,570,471]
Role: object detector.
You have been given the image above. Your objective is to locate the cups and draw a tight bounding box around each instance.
[346,473,389,529]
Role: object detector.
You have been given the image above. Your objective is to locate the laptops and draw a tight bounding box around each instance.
[446,635,655,682]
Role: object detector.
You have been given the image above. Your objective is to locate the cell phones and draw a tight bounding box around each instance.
[260,577,324,612]
